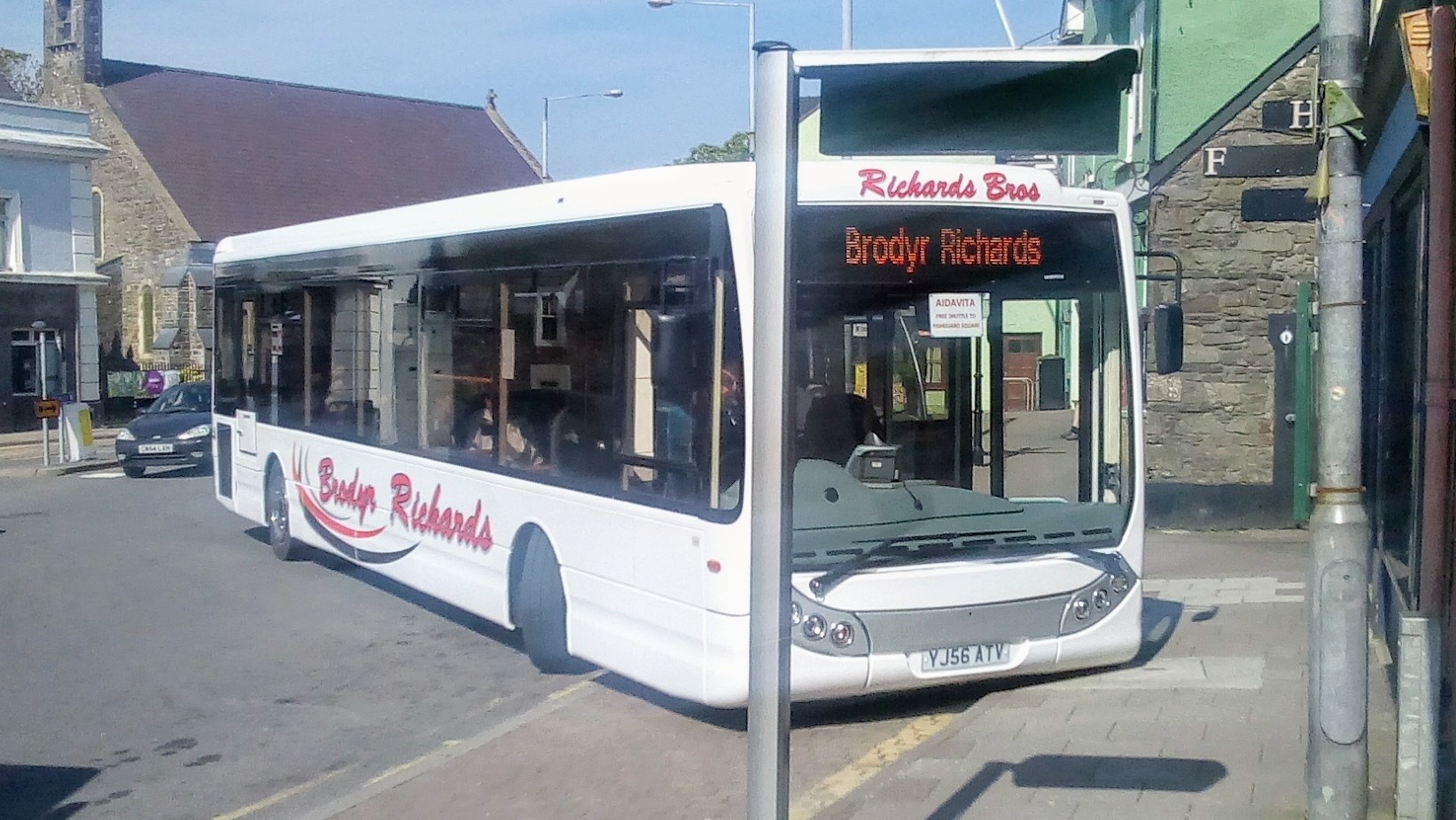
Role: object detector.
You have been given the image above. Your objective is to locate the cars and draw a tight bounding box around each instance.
[115,378,212,478]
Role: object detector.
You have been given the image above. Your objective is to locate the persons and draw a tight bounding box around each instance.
[458,385,548,472]
[693,357,745,487]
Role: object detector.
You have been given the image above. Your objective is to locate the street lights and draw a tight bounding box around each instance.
[648,1,756,161]
[541,89,624,182]
[32,321,51,466]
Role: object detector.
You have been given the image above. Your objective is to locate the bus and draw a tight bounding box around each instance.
[210,157,1184,712]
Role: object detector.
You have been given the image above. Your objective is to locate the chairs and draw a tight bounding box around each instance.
[321,400,700,498]
